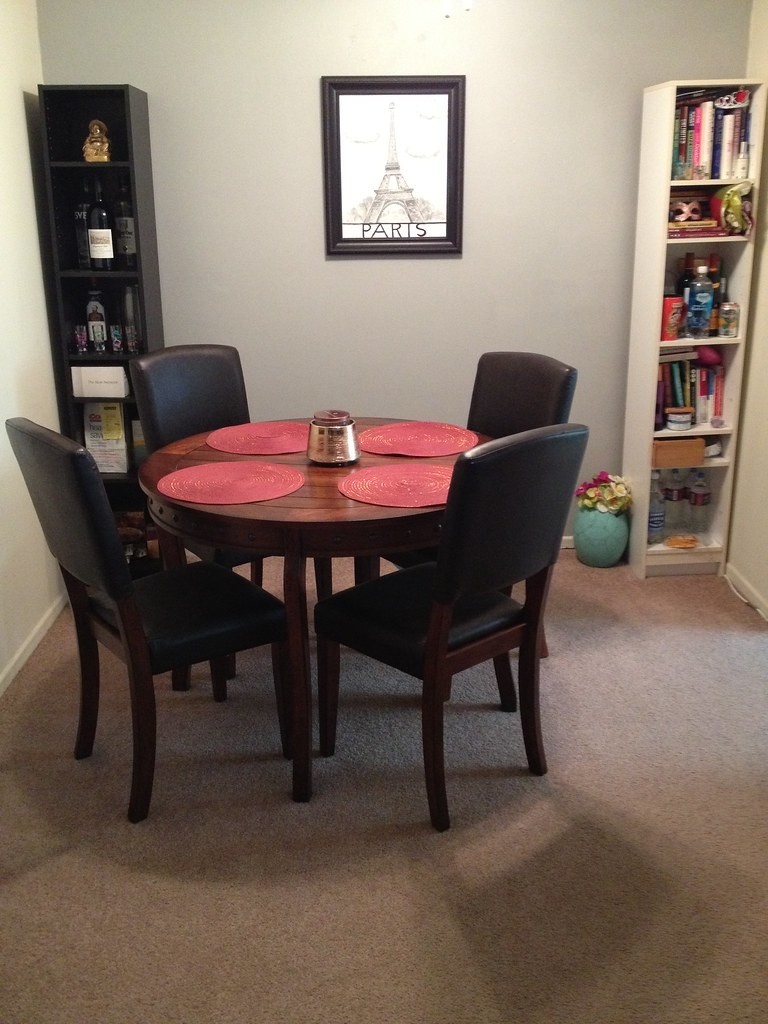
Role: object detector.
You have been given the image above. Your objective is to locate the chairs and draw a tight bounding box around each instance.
[356,352,581,658]
[309,425,586,835]
[129,347,288,678]
[8,417,288,821]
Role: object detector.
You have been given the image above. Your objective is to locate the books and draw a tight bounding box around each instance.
[671,88,750,179]
[668,221,729,238]
[654,346,724,431]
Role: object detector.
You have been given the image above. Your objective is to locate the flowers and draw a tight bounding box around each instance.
[573,471,635,514]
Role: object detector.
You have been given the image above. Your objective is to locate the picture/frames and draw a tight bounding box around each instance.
[322,74,464,254]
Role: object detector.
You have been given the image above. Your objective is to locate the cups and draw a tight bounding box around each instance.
[110,325,123,352]
[659,294,683,342]
[93,325,106,354]
[124,327,139,352]
[75,326,88,351]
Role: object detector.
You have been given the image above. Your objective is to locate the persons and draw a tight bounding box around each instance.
[82,125,109,152]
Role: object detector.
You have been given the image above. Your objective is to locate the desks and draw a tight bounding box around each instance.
[137,412,496,758]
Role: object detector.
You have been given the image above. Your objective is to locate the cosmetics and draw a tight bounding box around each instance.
[737,142,749,179]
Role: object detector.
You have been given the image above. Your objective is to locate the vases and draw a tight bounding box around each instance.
[573,507,631,569]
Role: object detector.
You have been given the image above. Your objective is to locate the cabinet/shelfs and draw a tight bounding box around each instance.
[36,82,163,451]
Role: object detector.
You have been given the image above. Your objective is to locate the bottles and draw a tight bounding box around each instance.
[86,176,116,270]
[686,264,714,339]
[676,252,695,337]
[84,279,111,349]
[113,174,138,271]
[707,256,721,336]
[734,141,750,181]
[73,175,95,269]
[117,285,142,341]
[649,469,714,542]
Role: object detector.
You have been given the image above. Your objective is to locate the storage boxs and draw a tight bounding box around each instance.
[68,363,136,475]
[651,437,706,468]
[622,82,767,576]
